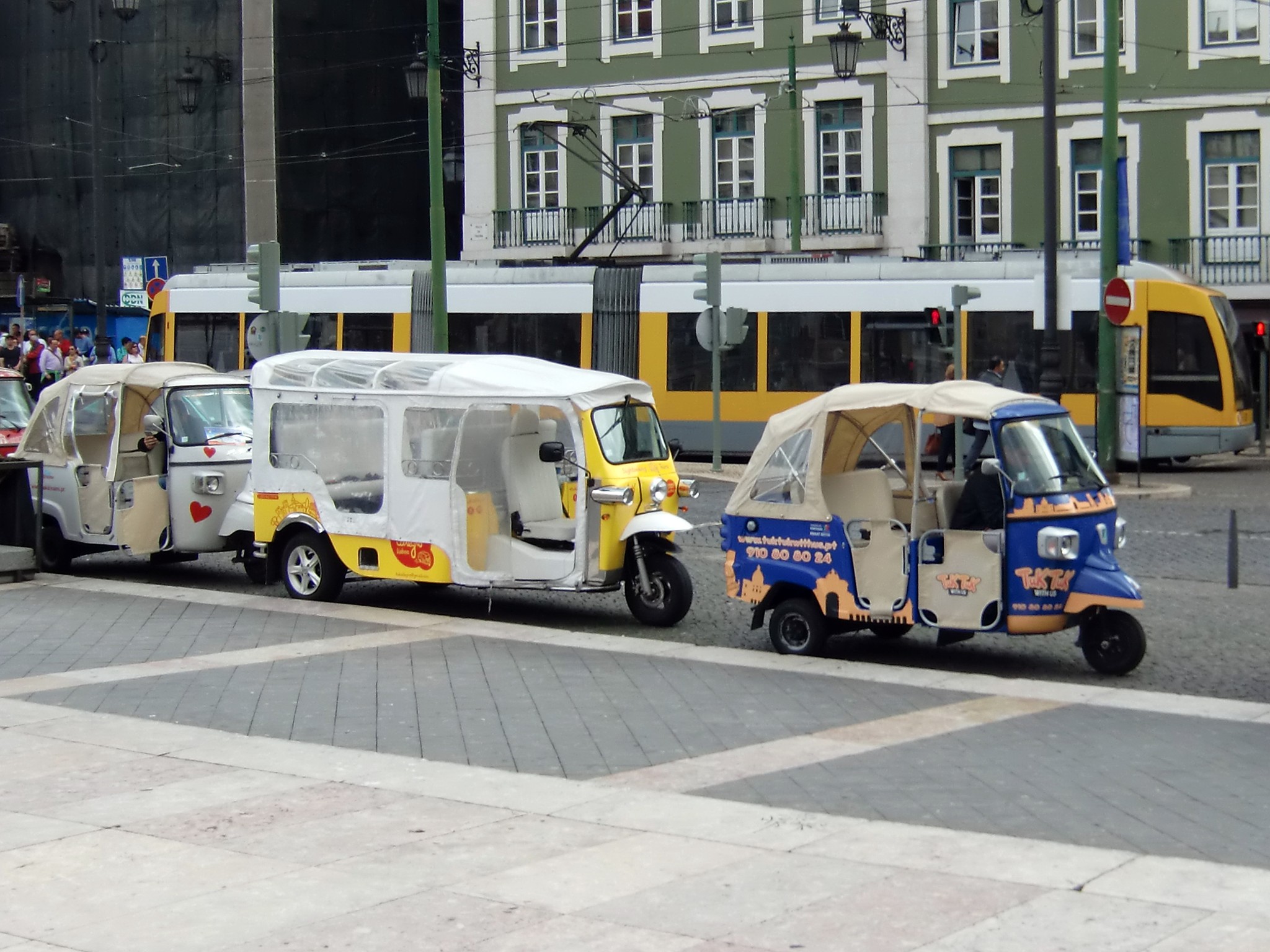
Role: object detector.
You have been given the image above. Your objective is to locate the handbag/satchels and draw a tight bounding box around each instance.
[962,417,977,436]
[925,426,942,455]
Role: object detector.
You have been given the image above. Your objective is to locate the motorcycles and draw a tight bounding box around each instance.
[249,349,704,629]
[7,361,258,573]
[0,366,35,461]
[719,380,1150,675]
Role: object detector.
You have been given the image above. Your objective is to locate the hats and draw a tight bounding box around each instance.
[82,329,90,334]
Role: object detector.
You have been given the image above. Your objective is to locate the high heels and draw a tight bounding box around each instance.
[936,472,950,481]
[950,468,955,478]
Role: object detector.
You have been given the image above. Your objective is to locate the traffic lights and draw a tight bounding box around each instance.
[923,306,948,347]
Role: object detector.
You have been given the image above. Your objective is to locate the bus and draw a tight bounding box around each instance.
[136,119,1262,469]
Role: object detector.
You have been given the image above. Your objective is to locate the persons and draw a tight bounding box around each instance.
[933,364,955,481]
[1030,388,1042,396]
[138,400,209,490]
[0,323,146,402]
[963,356,1005,478]
[950,427,1031,531]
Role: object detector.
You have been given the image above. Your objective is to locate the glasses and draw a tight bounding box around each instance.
[6,336,14,338]
[31,334,36,335]
[69,348,74,350]
[133,346,138,348]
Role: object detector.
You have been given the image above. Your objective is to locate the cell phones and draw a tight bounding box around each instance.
[144,430,155,444]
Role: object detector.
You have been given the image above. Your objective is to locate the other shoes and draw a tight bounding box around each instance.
[964,467,971,479]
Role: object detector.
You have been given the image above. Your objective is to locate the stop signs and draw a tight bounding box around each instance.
[1103,278,1131,327]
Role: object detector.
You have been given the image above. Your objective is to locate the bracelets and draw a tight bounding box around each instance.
[19,358,21,360]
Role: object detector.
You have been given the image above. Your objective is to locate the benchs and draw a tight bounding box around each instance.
[790,468,910,547]
[40,432,146,477]
[275,416,557,501]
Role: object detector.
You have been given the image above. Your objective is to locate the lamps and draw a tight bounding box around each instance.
[829,5,907,82]
[175,47,230,116]
[112,0,140,22]
[443,138,465,184]
[404,34,481,100]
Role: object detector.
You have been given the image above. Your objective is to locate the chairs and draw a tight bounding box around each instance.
[501,410,578,541]
[936,482,966,529]
